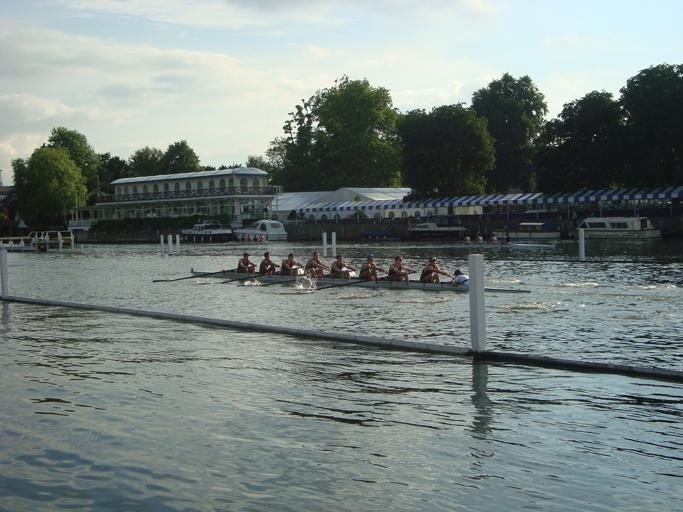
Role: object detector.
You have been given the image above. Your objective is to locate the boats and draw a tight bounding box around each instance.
[491,223,561,239]
[190,268,530,292]
[407,223,467,241]
[180,223,232,243]
[233,220,288,241]
[573,217,662,239]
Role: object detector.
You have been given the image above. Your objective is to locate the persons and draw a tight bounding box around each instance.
[303,252,329,278]
[503,232,511,245]
[246,235,250,242]
[259,252,279,274]
[475,233,483,244]
[488,234,497,245]
[387,256,414,282]
[236,253,256,274]
[240,233,245,241]
[252,234,257,242]
[330,255,356,279]
[280,253,303,275]
[463,234,470,244]
[452,269,469,287]
[258,233,263,241]
[419,256,449,283]
[358,254,386,281]
